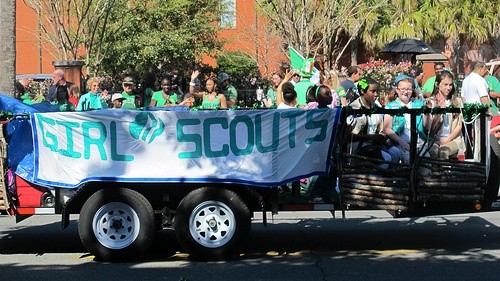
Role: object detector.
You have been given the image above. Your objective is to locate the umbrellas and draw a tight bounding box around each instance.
[379,38,436,61]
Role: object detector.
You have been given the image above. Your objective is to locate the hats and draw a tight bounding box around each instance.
[123,77,134,85]
[112,93,126,102]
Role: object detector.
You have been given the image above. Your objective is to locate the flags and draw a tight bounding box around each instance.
[288,45,322,85]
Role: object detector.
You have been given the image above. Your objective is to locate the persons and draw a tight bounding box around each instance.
[250,62,500,213]
[12,69,237,111]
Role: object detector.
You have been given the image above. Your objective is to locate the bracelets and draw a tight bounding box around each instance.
[188,82,197,88]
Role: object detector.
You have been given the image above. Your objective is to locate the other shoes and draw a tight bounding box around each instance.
[309,197,325,203]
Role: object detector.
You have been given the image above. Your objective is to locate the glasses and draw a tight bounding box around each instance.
[293,74,300,77]
[397,87,414,94]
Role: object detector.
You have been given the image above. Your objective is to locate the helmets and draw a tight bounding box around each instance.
[217,73,229,83]
